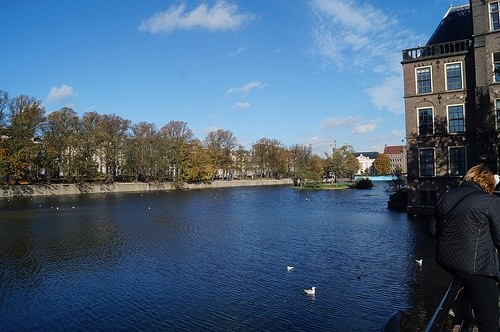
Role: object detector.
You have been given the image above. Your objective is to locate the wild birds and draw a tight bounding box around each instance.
[303,287,317,294]
[286,265,294,271]
[415,259,423,264]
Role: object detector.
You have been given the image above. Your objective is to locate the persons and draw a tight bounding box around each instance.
[436,165,500,332]
